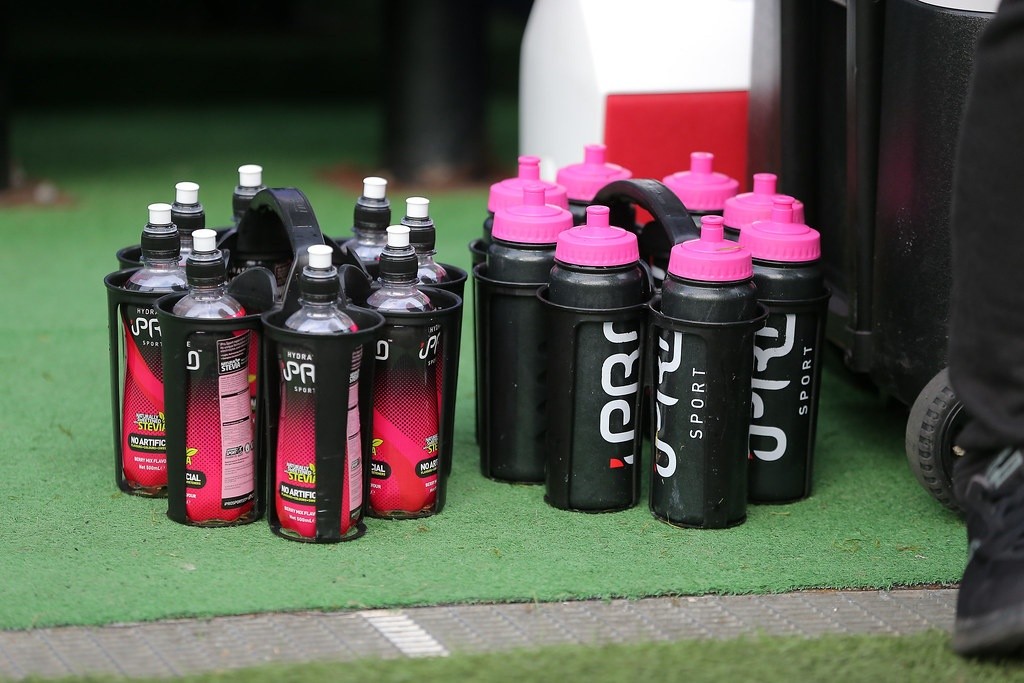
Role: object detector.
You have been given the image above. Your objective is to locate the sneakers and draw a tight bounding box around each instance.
[904,365,1023,663]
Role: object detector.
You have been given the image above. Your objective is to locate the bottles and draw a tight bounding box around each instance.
[463,142,827,531]
[120,163,449,538]
